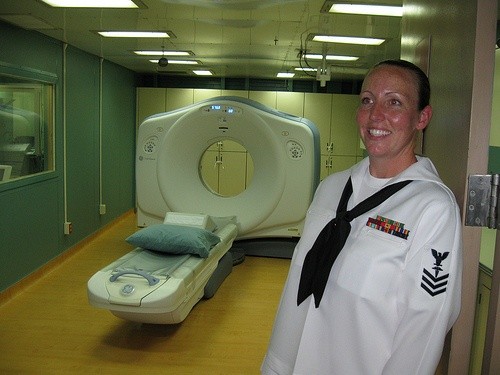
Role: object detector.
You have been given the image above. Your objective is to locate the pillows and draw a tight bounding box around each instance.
[124,224,221,258]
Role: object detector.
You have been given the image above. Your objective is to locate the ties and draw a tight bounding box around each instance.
[297,177,413,309]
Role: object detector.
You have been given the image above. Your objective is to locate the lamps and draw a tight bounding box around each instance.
[274,70,296,81]
[291,66,328,74]
[192,70,217,76]
[90,30,177,39]
[40,1,147,13]
[306,33,393,48]
[130,50,196,57]
[296,52,362,64]
[148,59,203,66]
[320,0,405,19]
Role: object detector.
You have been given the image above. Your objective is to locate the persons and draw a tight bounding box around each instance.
[263,60,462,375]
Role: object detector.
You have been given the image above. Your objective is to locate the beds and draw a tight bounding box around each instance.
[88,212,238,323]
